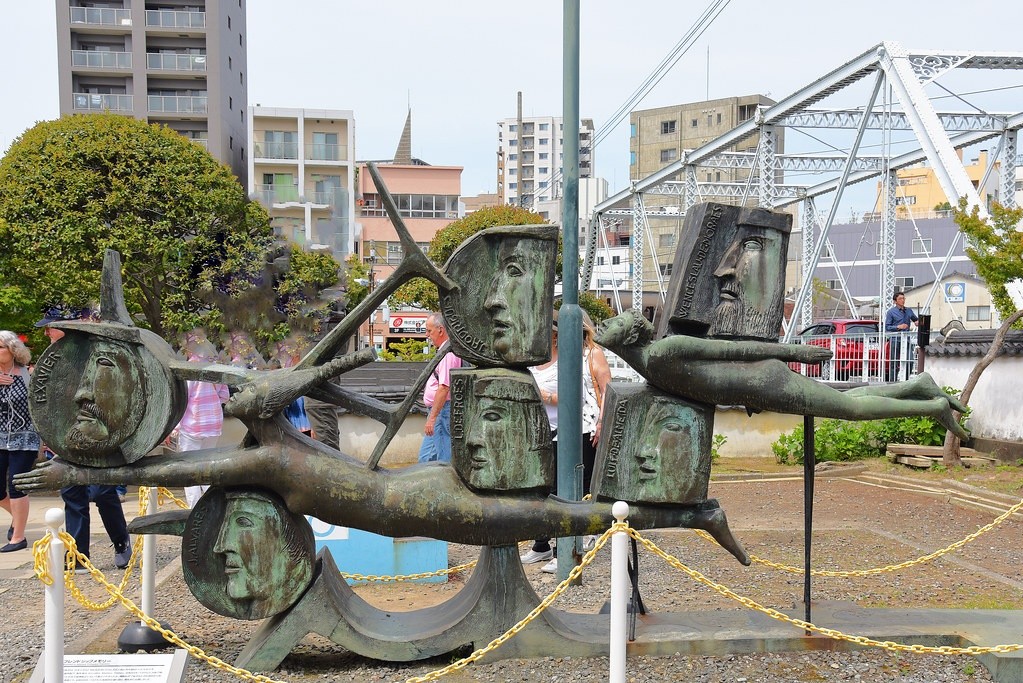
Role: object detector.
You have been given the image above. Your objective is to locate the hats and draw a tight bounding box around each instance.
[35,303,91,328]
[552,310,559,331]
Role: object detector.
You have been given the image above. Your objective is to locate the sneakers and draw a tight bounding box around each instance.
[520,550,552,564]
[115,535,132,569]
[64,562,88,574]
[541,558,557,573]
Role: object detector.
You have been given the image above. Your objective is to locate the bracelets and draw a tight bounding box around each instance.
[547,393,553,404]
[598,419,602,424]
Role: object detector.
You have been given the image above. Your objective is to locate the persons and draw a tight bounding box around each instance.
[484,237,549,357]
[164,330,230,508]
[11,349,752,569]
[709,226,782,339]
[591,307,972,445]
[465,381,547,489]
[34,305,132,570]
[634,400,706,501]
[61,338,144,453]
[884,293,920,382]
[0,330,40,552]
[212,488,307,601]
[417,313,461,463]
[223,332,313,438]
[520,307,613,575]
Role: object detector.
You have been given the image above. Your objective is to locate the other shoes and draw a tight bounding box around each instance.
[583,537,597,552]
[119,494,126,502]
[7,524,15,541]
[0,537,27,553]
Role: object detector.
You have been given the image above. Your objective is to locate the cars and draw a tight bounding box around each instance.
[784,319,900,381]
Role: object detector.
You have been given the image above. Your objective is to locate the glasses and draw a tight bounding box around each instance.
[895,297,906,300]
